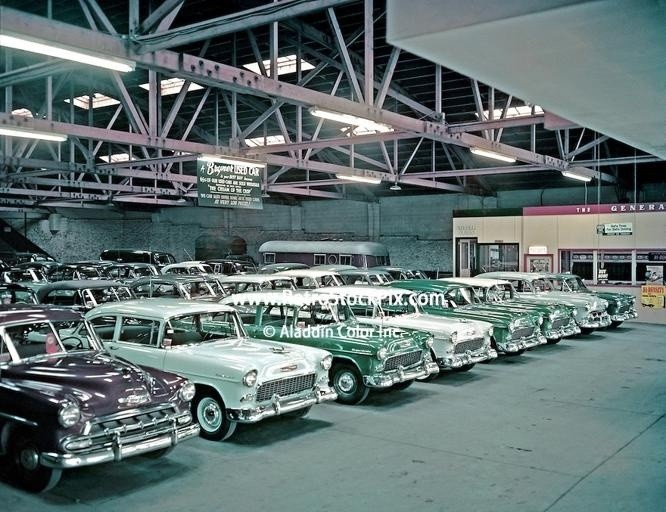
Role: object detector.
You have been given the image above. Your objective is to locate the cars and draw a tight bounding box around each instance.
[0,239,639,498]
